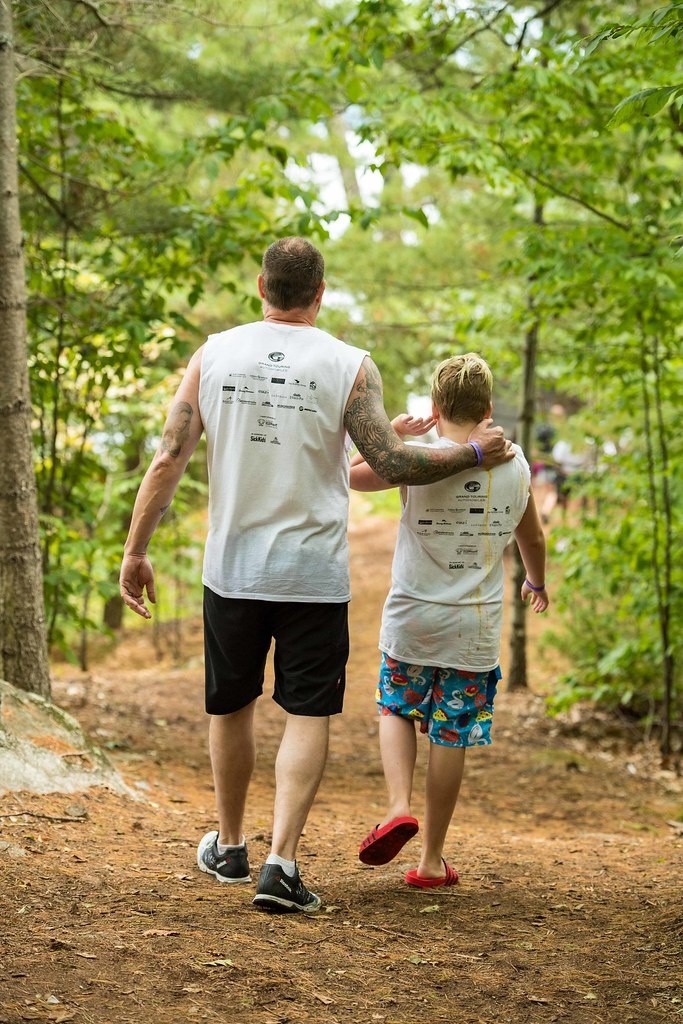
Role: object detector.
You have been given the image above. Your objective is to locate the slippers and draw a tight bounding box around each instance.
[405,857,459,888]
[359,816,419,866]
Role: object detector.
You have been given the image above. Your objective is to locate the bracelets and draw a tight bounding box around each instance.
[468,441,484,467]
[526,580,545,592]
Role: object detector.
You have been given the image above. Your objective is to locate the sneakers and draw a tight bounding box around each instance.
[251,859,321,913]
[197,831,252,884]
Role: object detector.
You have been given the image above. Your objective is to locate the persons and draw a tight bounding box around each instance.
[350,352,550,888]
[117,237,517,913]
